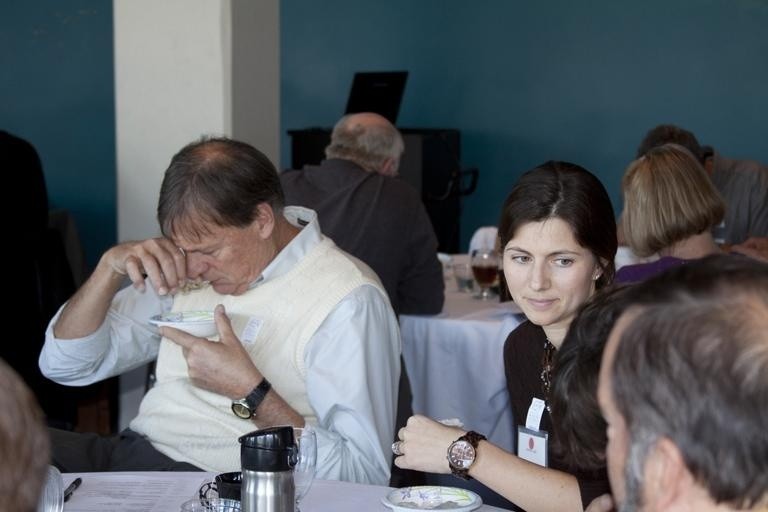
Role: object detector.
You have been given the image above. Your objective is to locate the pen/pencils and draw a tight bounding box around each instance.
[64,478,82,502]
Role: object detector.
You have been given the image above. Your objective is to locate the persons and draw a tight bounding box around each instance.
[2,131,83,430]
[391,161,611,511]
[548,253,768,472]
[596,293,768,511]
[1,366,49,512]
[38,137,403,484]
[283,112,445,316]
[614,127,768,288]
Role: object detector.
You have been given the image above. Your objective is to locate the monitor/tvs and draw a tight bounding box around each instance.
[346,70,411,126]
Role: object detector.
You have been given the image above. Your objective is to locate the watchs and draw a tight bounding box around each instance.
[445,430,487,481]
[231,377,272,419]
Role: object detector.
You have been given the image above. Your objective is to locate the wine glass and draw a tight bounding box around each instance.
[287,429,317,512]
[470,249,500,302]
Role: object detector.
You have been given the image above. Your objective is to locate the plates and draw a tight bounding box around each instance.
[149,310,218,338]
[382,485,482,512]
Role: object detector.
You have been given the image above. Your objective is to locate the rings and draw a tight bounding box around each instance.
[391,441,402,456]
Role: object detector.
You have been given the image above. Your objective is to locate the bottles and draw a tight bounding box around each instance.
[241,427,301,512]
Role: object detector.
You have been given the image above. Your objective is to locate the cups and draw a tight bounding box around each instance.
[453,263,474,294]
[217,473,241,503]
